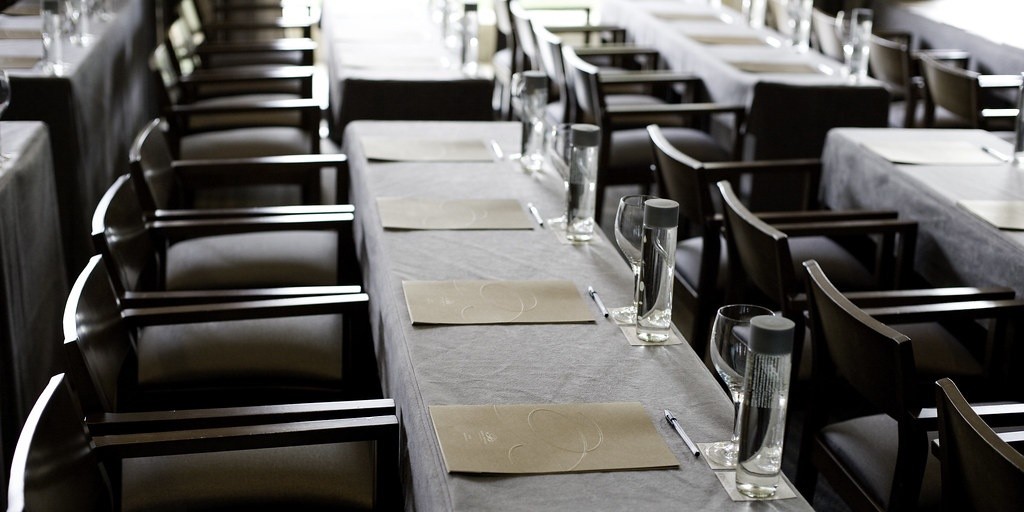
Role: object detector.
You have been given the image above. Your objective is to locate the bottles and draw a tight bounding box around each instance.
[637,201,681,345]
[567,122,602,244]
[850,9,873,81]
[737,317,795,499]
[521,71,549,155]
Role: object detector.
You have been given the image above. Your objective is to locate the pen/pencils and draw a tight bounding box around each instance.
[588,285,609,318]
[490,138,504,159]
[528,202,543,226]
[982,144,1009,162]
[664,409,700,457]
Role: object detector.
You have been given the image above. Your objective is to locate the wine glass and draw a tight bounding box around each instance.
[0,67,12,163]
[834,8,857,76]
[545,124,569,230]
[614,193,660,326]
[509,73,542,143]
[704,304,777,469]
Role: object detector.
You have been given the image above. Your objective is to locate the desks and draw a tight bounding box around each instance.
[818,130,1023,310]
[0,0,147,228]
[340,120,817,512]
[1,121,69,462]
[321,0,496,147]
[599,2,906,149]
[876,1,1024,103]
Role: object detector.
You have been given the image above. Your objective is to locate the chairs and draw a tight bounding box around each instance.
[484,0,1023,512]
[4,1,420,512]
